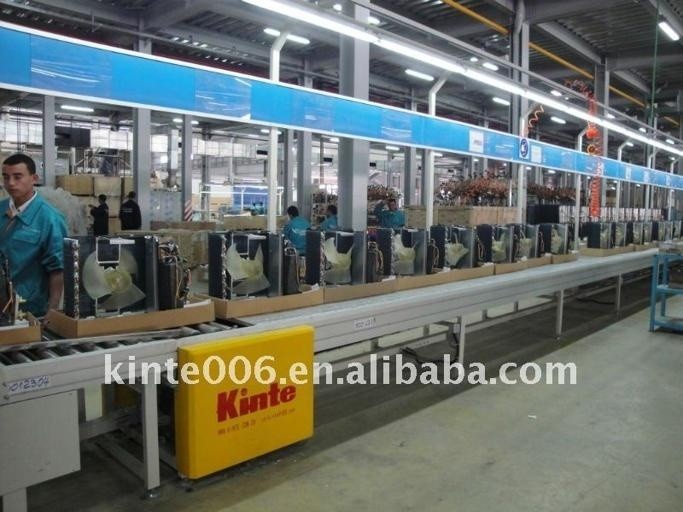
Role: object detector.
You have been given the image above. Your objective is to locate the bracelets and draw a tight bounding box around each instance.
[47,307,56,313]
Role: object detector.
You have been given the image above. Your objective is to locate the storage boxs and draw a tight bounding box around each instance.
[55,174,134,235]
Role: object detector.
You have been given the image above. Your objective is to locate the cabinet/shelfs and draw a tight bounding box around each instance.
[649,254,683,334]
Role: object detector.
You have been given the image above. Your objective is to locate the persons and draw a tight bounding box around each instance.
[258,201,264,213]
[251,203,258,211]
[86,194,108,237]
[119,191,141,231]
[283,206,313,255]
[320,205,335,230]
[0,154,70,328]
[373,199,405,234]
